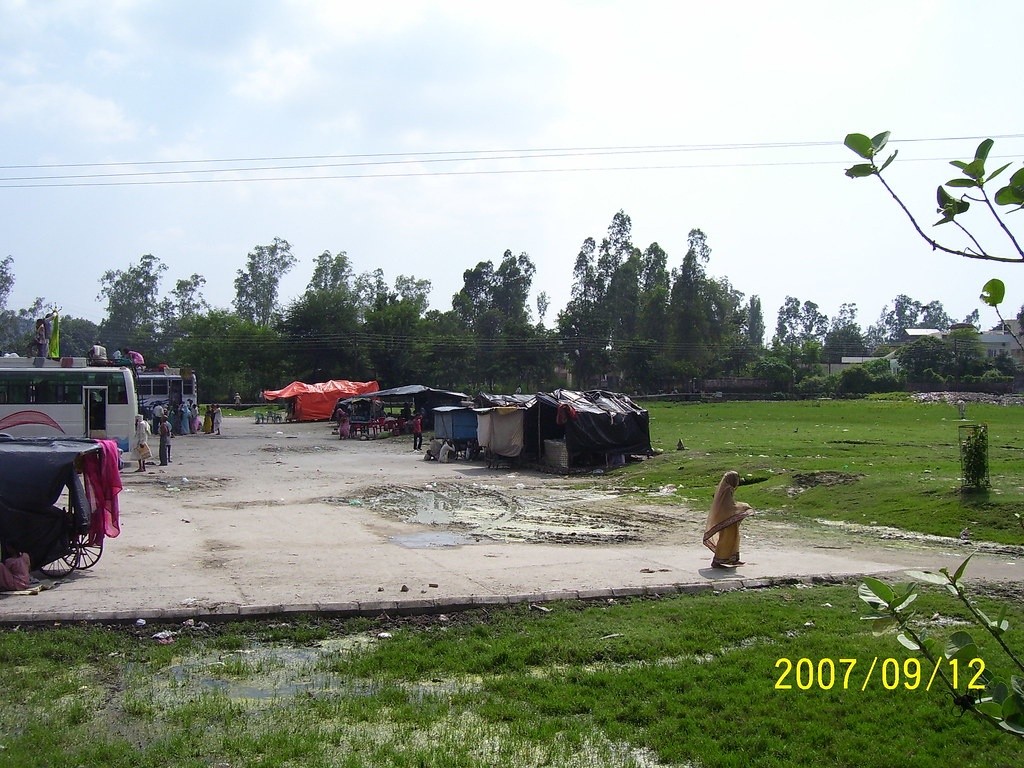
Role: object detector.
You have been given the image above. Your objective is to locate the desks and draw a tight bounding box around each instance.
[352,422,380,435]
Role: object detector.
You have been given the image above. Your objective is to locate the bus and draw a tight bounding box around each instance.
[135,368,198,421]
[0,353,138,461]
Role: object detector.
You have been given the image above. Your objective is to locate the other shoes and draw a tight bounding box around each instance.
[135,468,145,472]
[160,463,168,466]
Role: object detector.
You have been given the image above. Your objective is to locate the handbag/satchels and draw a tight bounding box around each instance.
[136,443,152,459]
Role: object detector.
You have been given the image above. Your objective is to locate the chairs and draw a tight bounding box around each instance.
[254,411,282,424]
[379,417,406,433]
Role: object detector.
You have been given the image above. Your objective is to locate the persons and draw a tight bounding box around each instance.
[515,384,523,394]
[113,347,144,364]
[158,415,173,466]
[132,414,152,472]
[32,312,58,368]
[412,413,422,450]
[336,408,350,441]
[159,359,169,372]
[402,402,411,421]
[234,392,242,406]
[427,437,455,463]
[91,392,97,416]
[703,470,751,568]
[87,341,108,363]
[153,399,202,438]
[203,404,222,435]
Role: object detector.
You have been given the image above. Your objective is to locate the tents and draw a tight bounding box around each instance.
[473,387,652,466]
[262,379,380,421]
[362,384,469,431]
